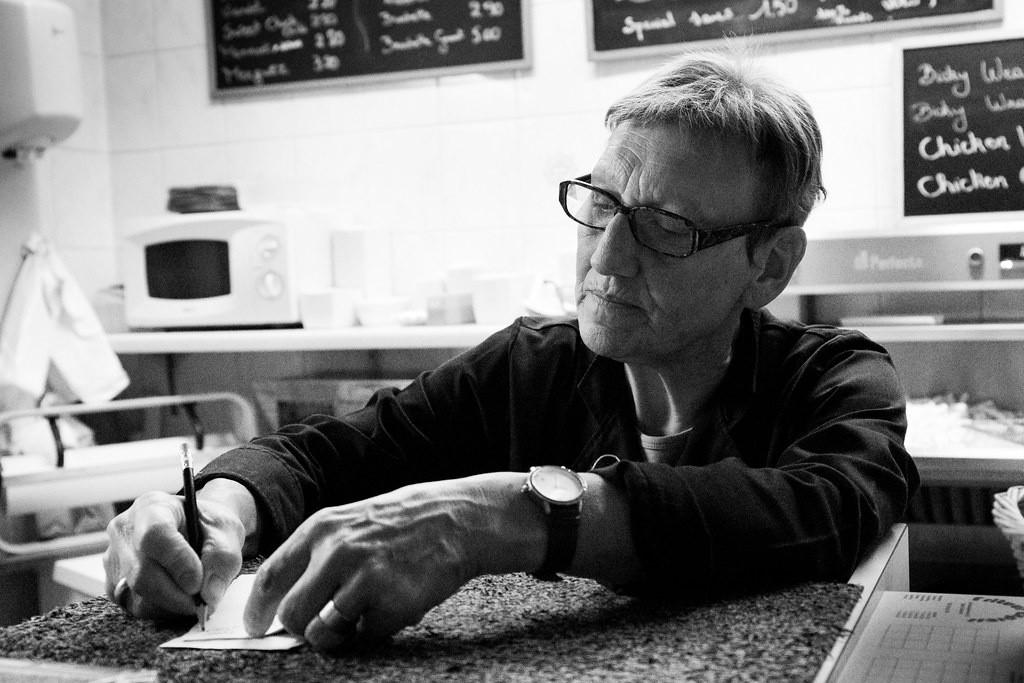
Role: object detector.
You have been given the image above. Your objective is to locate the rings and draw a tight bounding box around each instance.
[114,577,129,605]
[319,600,351,631]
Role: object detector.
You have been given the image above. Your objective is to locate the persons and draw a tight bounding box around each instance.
[101,56,921,654]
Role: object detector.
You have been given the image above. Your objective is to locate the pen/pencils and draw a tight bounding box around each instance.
[178,443,208,630]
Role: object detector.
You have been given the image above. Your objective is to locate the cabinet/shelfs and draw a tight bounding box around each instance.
[771,281,1024,486]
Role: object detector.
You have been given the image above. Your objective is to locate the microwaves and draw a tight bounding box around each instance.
[117,209,333,332]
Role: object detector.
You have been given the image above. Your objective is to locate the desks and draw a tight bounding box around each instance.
[96,323,498,411]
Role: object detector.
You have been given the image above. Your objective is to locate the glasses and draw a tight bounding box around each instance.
[559,174,798,257]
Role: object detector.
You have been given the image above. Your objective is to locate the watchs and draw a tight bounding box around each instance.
[521,465,587,583]
